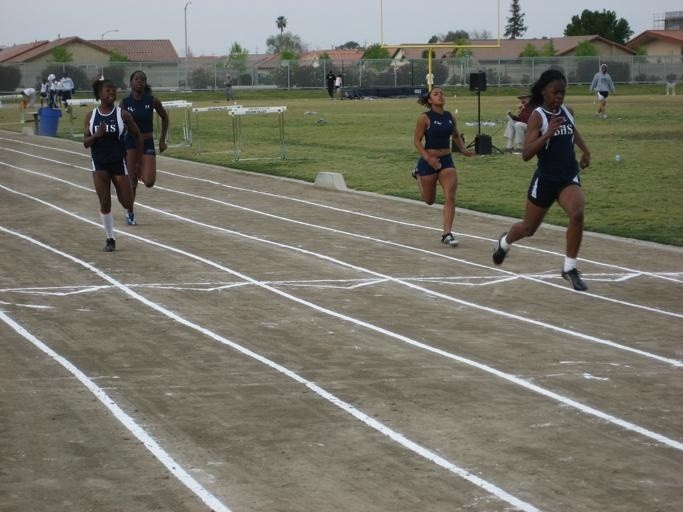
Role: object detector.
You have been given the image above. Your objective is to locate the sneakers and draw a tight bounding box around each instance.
[492,232,510,264]
[440,233,459,247]
[512,148,523,154]
[498,148,514,153]
[126,211,136,226]
[106,238,115,252]
[562,268,588,291]
[411,167,419,178]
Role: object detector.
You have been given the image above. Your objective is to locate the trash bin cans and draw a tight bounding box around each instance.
[39,108,62,137]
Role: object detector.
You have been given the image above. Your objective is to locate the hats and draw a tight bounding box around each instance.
[518,92,532,98]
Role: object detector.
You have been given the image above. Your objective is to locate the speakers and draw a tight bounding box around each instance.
[470,72,487,91]
[475,134,492,154]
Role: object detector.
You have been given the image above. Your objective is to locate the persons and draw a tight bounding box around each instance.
[493,69,591,291]
[412,87,475,247]
[83,79,144,253]
[326,70,336,97]
[224,74,234,101]
[426,73,435,87]
[502,91,534,154]
[334,73,342,87]
[589,63,616,119]
[22,73,75,112]
[119,71,169,202]
